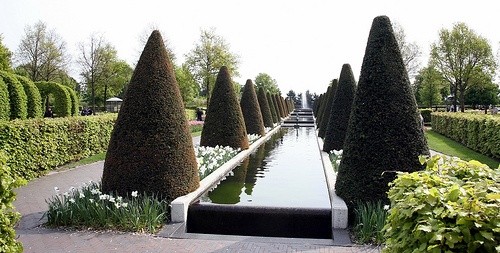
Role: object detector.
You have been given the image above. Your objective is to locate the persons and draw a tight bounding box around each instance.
[42,105,93,119]
[195,108,205,121]
